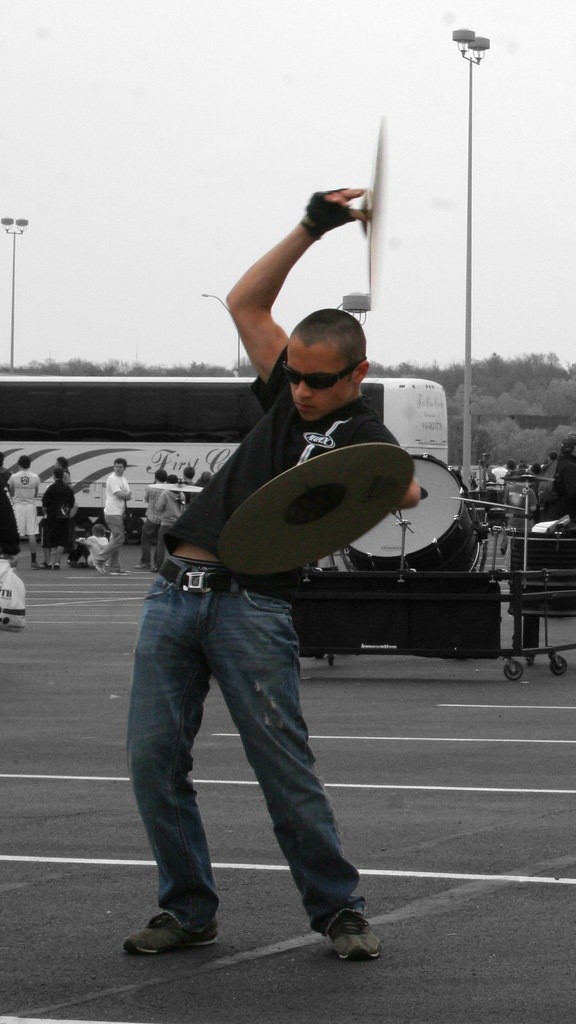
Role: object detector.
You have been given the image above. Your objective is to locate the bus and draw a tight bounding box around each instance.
[0,376,448,545]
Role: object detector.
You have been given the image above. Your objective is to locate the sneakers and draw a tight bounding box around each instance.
[326,908,381,959]
[123,912,218,954]
[93,560,108,574]
[110,569,131,575]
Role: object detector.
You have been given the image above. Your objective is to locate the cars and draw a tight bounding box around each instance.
[450,465,504,504]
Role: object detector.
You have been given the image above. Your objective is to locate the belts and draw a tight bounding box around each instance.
[160,557,231,593]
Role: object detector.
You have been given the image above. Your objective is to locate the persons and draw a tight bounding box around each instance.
[0,452,131,575]
[134,467,213,570]
[123,188,429,960]
[485,433,576,554]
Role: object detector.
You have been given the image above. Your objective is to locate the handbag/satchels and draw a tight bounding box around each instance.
[0,560,26,633]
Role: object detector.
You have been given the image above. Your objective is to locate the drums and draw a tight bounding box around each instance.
[343,452,482,573]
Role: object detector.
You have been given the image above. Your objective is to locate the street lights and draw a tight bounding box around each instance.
[0,217,28,372]
[201,293,241,376]
[452,27,492,483]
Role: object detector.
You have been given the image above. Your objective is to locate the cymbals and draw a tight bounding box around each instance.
[148,482,204,493]
[499,473,556,484]
[367,114,391,298]
[448,495,525,513]
[216,440,416,576]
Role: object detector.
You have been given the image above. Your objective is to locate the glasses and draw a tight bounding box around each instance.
[282,357,367,390]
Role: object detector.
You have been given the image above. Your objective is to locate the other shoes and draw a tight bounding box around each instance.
[39,562,52,569]
[54,565,60,570]
[134,564,150,569]
[31,562,38,570]
